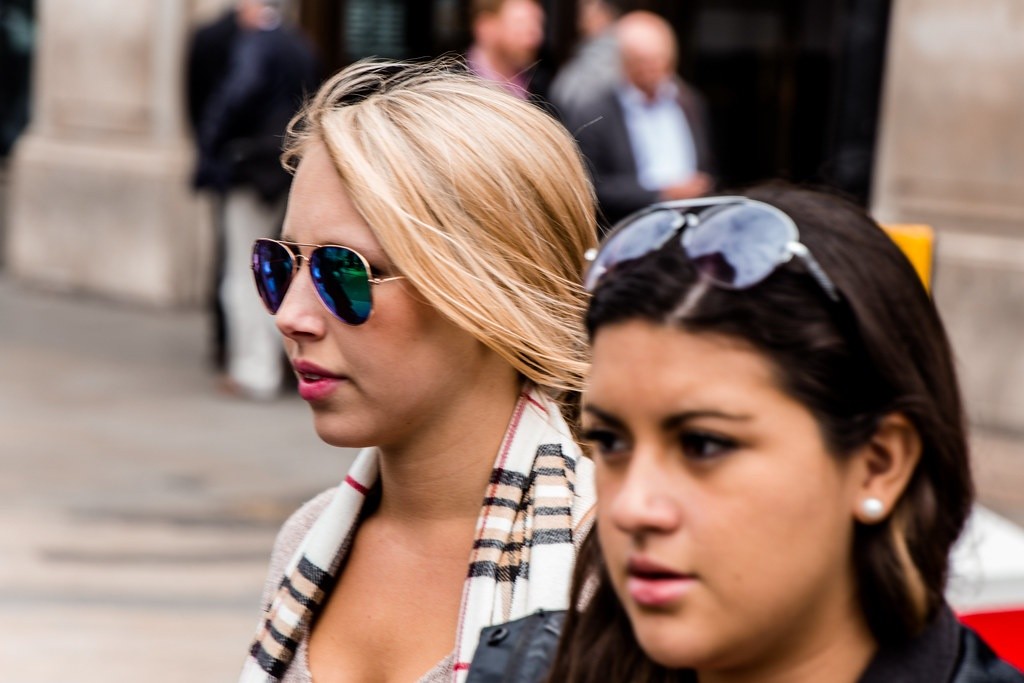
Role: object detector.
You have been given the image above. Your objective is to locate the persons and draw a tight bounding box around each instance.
[465,0,716,217]
[0,0,42,259]
[235,59,598,683]
[179,0,424,397]
[462,183,1024,683]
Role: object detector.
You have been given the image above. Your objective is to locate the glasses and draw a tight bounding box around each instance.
[586,196,842,312]
[251,236,413,327]
